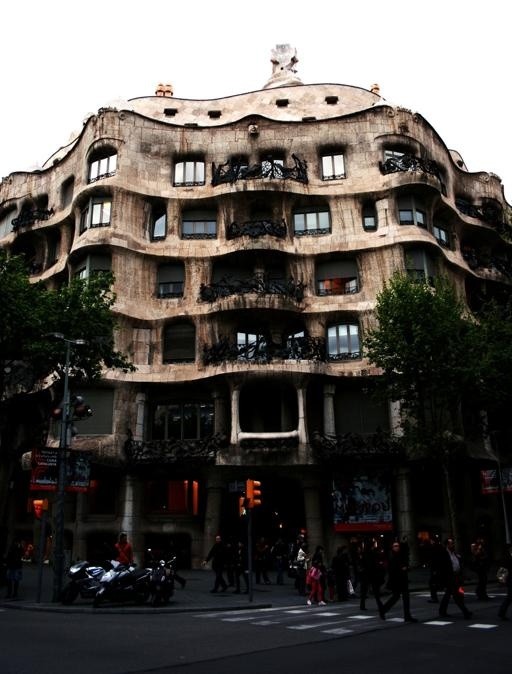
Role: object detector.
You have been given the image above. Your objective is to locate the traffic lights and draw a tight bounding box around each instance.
[245,478,262,508]
[51,402,92,420]
[33,498,49,519]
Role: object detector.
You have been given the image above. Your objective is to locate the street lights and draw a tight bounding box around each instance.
[48,332,89,603]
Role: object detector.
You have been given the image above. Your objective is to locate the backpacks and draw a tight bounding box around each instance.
[306,571,312,585]
[429,556,445,587]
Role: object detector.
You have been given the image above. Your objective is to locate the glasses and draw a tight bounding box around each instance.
[392,545,401,547]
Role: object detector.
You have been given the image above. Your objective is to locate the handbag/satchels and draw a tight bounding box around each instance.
[289,561,304,579]
[347,580,355,594]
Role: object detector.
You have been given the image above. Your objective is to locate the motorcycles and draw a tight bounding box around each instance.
[57,548,177,607]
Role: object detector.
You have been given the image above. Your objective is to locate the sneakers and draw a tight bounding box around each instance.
[319,601,326,605]
[306,600,312,605]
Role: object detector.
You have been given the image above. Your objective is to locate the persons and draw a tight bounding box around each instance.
[378,541,418,623]
[421,536,512,619]
[255,537,384,610]
[115,531,135,570]
[202,537,249,594]
